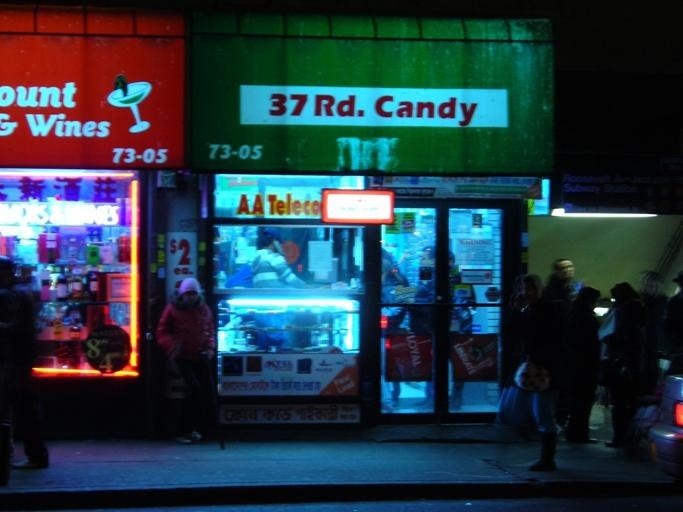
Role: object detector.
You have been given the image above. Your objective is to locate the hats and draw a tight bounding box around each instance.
[179,277,199,295]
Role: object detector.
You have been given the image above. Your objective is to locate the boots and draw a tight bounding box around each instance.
[528,429,557,471]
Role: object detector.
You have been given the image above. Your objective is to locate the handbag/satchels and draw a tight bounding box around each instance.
[514,361,548,392]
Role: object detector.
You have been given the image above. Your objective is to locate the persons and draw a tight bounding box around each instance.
[407,245,478,412]
[251,227,307,289]
[505,255,682,472]
[380,247,410,401]
[154,279,215,447]
[0,255,54,490]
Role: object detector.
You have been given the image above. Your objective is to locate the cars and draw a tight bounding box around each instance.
[647,374,682,481]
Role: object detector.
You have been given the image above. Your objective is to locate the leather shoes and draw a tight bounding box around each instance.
[10,457,46,468]
[174,430,201,444]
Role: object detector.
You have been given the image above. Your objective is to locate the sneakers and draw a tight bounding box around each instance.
[419,397,462,411]
[564,435,597,443]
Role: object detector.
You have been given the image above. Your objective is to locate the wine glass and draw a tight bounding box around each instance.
[106,80,153,134]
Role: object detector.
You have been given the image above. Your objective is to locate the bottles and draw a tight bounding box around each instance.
[52,316,63,334]
[68,311,82,340]
[39,265,97,302]
